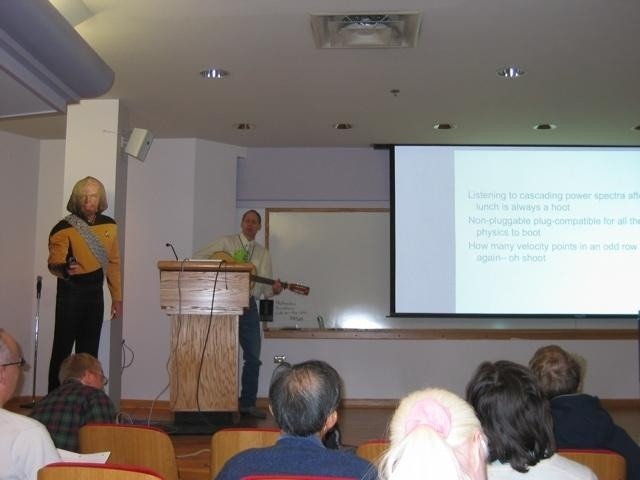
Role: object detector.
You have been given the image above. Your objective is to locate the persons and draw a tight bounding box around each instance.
[215,360,376,480]
[191,210,282,418]
[363,389,488,480]
[27,353,116,452]
[469,359,599,480]
[529,346,640,480]
[0,328,64,480]
[47,177,122,393]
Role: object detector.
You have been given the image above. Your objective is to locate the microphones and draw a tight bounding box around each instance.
[166,243,179,261]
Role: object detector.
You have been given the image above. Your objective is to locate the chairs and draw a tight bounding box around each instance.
[79,422,181,480]
[358,440,390,472]
[38,462,160,479]
[554,447,626,479]
[211,427,284,477]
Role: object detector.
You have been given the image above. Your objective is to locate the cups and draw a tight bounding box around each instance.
[233,249,247,264]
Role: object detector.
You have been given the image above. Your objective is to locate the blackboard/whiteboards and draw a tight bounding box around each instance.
[262,208,640,340]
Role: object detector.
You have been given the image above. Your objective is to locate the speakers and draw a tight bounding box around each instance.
[260,300,274,321]
[124,127,154,161]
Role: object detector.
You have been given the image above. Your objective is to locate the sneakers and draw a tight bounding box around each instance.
[239,405,267,418]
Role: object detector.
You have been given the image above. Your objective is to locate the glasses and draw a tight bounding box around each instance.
[89,370,108,385]
[0,357,25,367]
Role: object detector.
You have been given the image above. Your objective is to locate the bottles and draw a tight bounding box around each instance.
[317,316,325,329]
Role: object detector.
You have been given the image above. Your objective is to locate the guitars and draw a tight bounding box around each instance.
[208,251,309,295]
